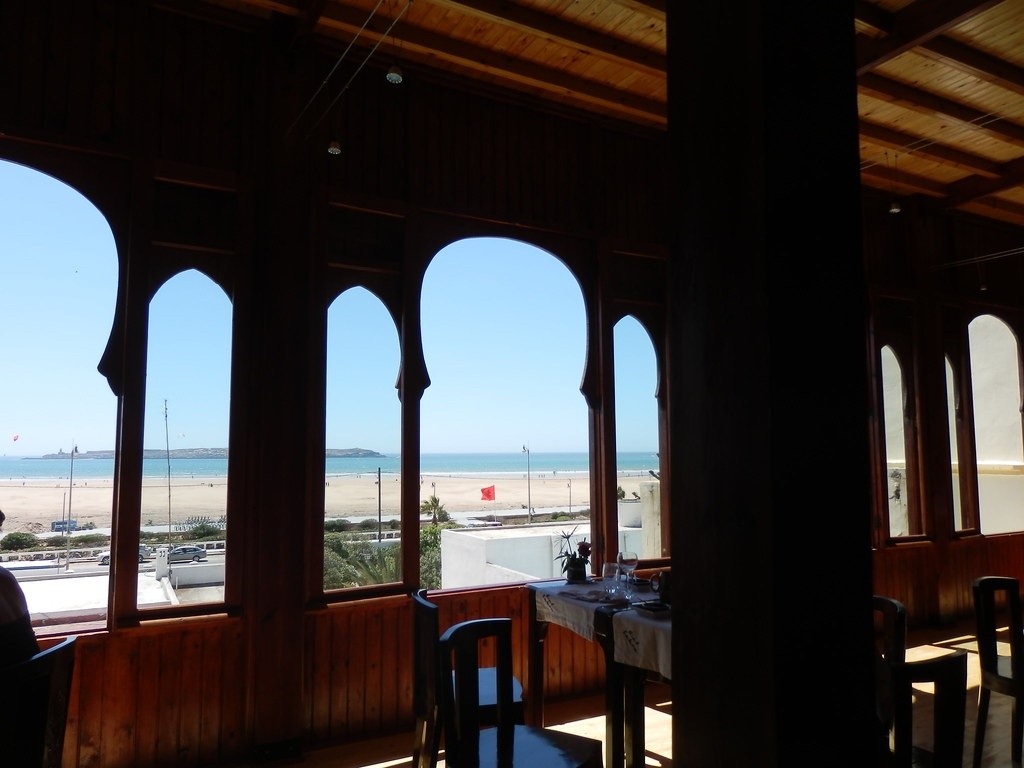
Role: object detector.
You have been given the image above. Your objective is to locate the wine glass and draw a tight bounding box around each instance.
[617,551,639,599]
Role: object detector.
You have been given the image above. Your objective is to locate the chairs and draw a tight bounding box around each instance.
[411,588,526,767]
[872,595,967,767]
[434,618,604,767]
[0,636,77,767]
[971,575,1024,768]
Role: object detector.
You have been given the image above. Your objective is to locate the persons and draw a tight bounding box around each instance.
[0,504,48,768]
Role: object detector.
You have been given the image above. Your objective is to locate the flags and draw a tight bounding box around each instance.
[481,485,495,501]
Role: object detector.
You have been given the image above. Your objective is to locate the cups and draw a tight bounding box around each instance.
[649,571,678,612]
[601,563,621,597]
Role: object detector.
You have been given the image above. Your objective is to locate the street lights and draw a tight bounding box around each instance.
[65,445,79,571]
[522,446,533,526]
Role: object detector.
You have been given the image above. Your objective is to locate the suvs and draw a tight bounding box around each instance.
[95,544,151,566]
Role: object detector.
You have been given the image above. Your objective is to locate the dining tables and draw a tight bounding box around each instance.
[612,603,674,768]
[525,574,661,768]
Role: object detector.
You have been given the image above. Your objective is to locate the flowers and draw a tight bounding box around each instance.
[555,532,594,573]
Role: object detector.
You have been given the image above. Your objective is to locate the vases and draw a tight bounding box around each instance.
[567,558,587,584]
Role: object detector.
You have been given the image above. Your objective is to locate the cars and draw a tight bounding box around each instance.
[165,546,207,565]
